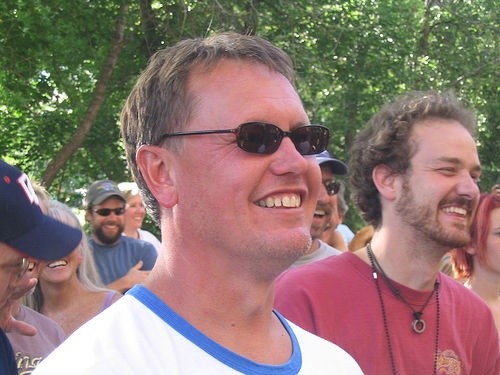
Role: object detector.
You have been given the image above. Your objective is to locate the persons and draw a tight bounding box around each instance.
[0,93,500,375]
[32,32,375,375]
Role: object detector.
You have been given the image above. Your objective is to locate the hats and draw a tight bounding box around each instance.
[311,150,348,176]
[0,160,82,261]
[87,180,127,209]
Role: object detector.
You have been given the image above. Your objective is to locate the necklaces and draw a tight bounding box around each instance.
[367,244,436,334]
[366,243,439,375]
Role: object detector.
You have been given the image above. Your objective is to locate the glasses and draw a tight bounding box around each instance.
[0,256,30,281]
[156,122,330,155]
[91,208,126,216]
[324,179,341,196]
